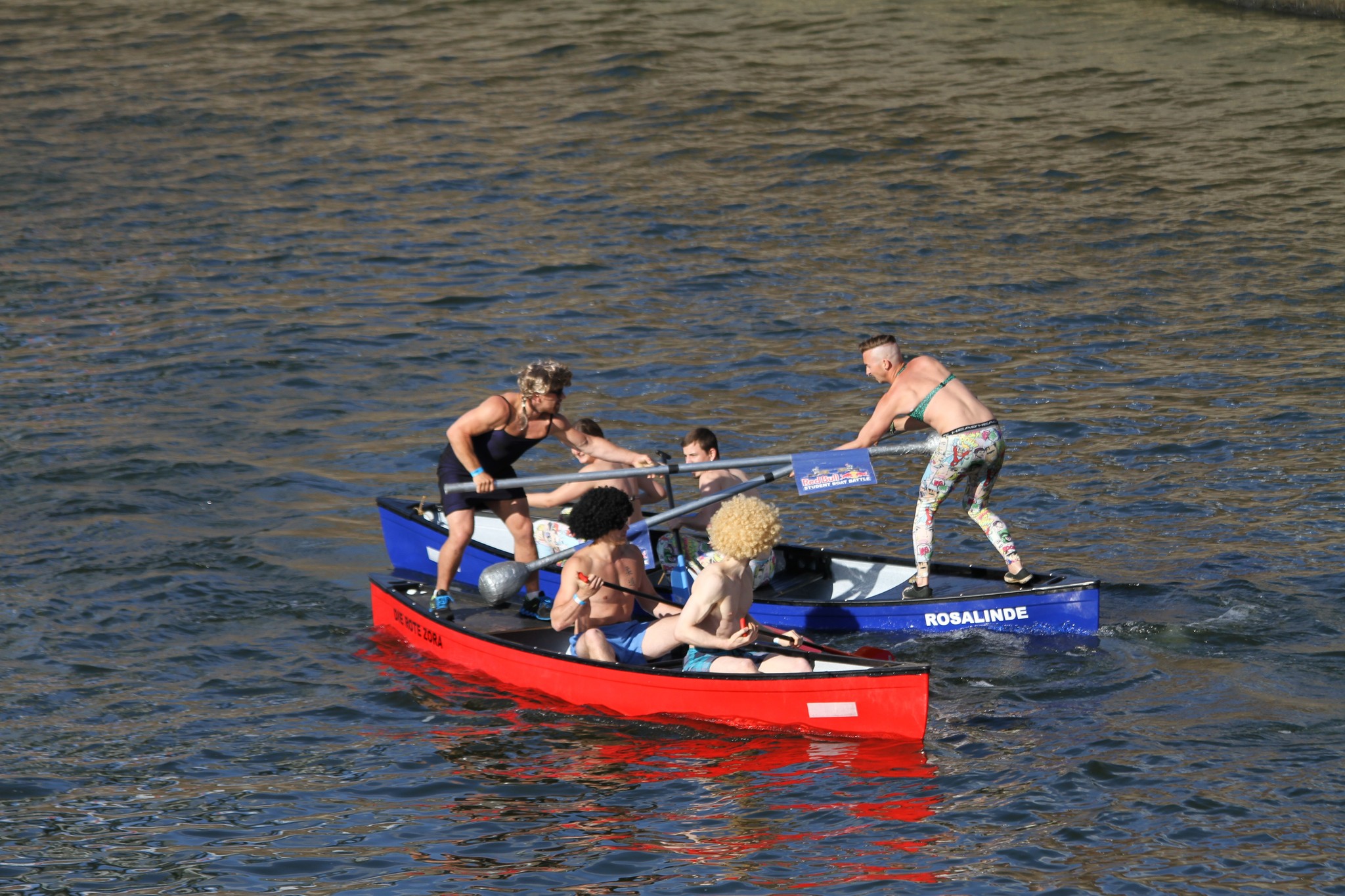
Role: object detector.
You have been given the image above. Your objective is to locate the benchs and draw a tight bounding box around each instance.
[636,551,832,669]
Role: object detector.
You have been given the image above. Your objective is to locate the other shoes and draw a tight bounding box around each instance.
[902,580,931,599]
[1004,568,1033,584]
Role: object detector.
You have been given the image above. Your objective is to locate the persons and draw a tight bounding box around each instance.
[656,428,777,592]
[674,494,812,673]
[549,485,685,664]
[429,359,664,621]
[525,417,667,572]
[787,333,1033,599]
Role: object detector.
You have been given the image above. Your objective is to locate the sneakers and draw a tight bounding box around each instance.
[519,591,554,620]
[428,589,457,619]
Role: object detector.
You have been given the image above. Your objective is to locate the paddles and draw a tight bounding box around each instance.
[578,572,822,653]
[740,618,896,660]
[558,502,699,518]
[655,448,695,605]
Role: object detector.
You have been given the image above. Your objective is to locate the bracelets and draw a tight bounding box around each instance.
[470,467,485,478]
[574,594,587,605]
[773,637,778,644]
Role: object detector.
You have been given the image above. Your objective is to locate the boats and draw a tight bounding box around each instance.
[375,494,1101,639]
[367,568,931,745]
